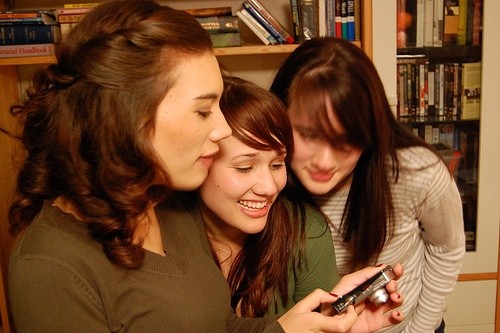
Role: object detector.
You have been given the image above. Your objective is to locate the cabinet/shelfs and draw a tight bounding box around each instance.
[0,0,373,282]
[372,0,500,333]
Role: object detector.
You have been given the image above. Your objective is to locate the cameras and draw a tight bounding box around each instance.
[331,264,397,315]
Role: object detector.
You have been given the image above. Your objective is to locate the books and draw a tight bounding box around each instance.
[1,1,483,253]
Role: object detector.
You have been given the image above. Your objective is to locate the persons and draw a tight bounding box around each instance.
[9,1,465,331]
